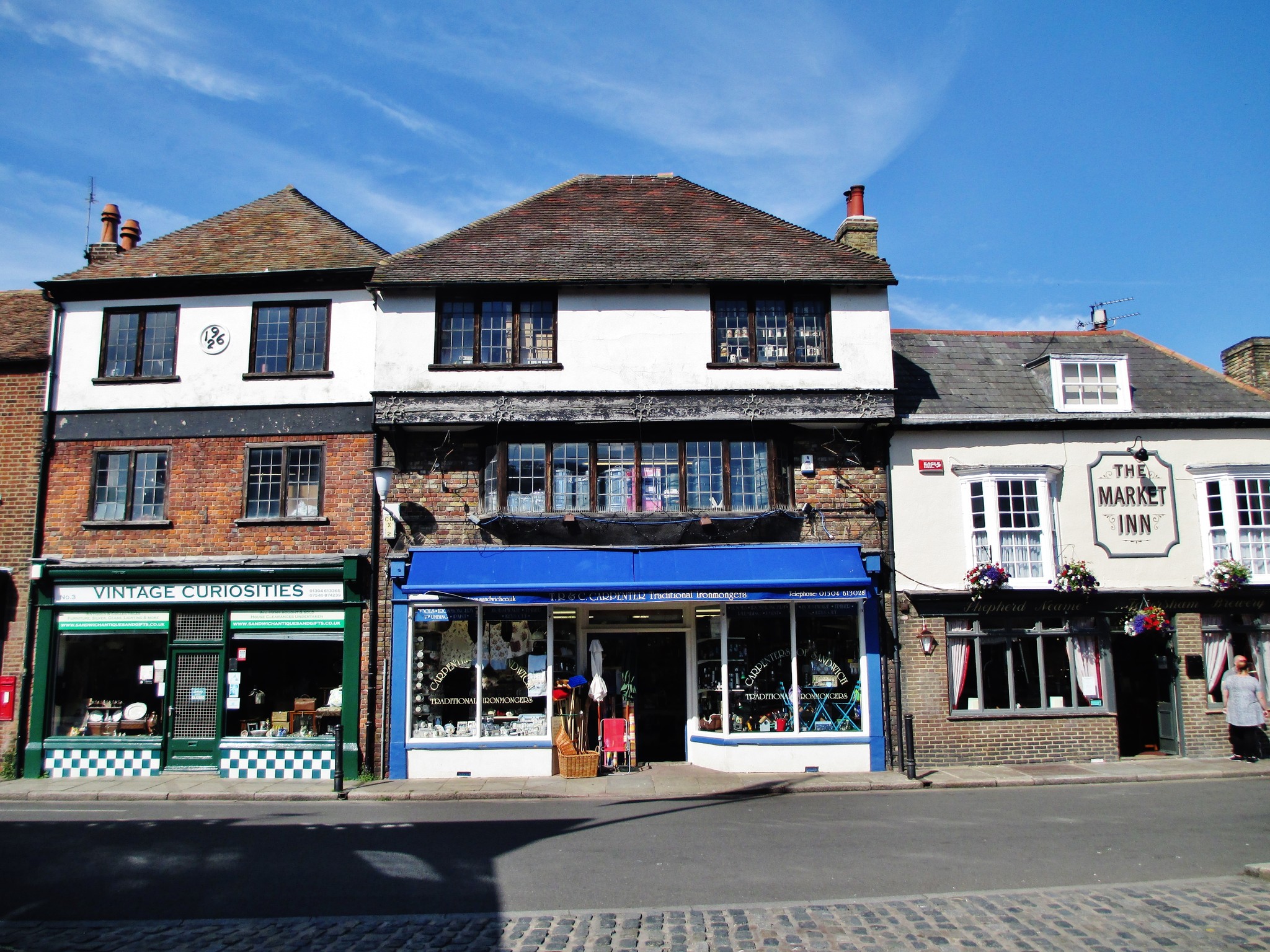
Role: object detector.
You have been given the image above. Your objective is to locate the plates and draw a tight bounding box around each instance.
[88,710,103,722]
[124,702,147,720]
[444,724,455,734]
[433,724,444,731]
[112,710,122,722]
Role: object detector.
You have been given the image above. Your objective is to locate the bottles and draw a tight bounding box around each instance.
[531,629,544,639]
[728,667,746,690]
[808,640,815,649]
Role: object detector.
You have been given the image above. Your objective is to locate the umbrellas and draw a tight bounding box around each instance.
[588,639,608,752]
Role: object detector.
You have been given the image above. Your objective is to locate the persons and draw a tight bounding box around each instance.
[1220,655,1270,763]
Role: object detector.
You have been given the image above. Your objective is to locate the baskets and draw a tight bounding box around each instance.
[557,746,600,778]
[555,724,578,755]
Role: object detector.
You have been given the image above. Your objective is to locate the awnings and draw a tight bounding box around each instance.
[400,548,872,604]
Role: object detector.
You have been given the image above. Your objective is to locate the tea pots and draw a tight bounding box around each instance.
[416,720,428,728]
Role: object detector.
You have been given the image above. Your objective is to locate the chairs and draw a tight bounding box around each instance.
[780,681,809,731]
[832,680,861,731]
[598,719,630,775]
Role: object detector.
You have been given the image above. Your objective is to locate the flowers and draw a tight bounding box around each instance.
[1048,557,1105,594]
[1124,606,1170,637]
[963,562,1011,602]
[1193,559,1253,592]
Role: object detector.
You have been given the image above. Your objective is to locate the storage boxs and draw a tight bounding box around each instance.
[506,305,552,363]
[272,722,290,733]
[272,711,290,722]
[812,675,837,686]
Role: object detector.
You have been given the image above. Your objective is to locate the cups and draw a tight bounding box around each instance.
[412,636,439,722]
[430,730,446,737]
[413,724,420,730]
[426,722,433,729]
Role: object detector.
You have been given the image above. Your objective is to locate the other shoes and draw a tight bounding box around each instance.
[1230,756,1242,762]
[1247,758,1255,763]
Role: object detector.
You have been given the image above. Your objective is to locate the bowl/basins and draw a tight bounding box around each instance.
[250,730,267,736]
[241,730,248,737]
[456,730,466,735]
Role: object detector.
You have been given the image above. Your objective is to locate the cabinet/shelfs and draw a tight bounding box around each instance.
[530,639,574,672]
[697,637,745,693]
[722,336,821,359]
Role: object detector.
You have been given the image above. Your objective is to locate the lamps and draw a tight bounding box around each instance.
[916,629,934,653]
[1127,436,1148,461]
[366,466,400,508]
[564,512,575,521]
[699,514,712,526]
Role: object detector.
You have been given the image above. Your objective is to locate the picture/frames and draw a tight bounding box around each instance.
[247,722,258,737]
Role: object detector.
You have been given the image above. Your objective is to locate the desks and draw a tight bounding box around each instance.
[289,711,316,734]
[801,687,838,731]
